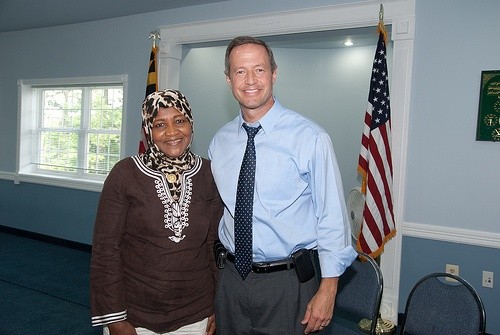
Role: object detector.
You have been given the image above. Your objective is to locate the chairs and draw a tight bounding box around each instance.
[315,251,384,335]
[396,272,489,335]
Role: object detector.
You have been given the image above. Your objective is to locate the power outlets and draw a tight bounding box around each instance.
[482,271,494,289]
[445,264,459,282]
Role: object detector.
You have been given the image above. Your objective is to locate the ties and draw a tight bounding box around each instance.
[234,123,262,281]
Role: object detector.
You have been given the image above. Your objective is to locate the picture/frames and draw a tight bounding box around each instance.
[475,69,500,143]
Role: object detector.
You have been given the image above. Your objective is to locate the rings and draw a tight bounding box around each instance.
[320,324,325,327]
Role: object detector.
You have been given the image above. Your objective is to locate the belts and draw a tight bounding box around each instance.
[228,252,294,275]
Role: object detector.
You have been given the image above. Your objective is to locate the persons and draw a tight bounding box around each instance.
[90,88,230,335]
[201,35,359,335]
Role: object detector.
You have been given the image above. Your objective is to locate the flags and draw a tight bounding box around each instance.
[139,47,158,154]
[357,20,396,262]
